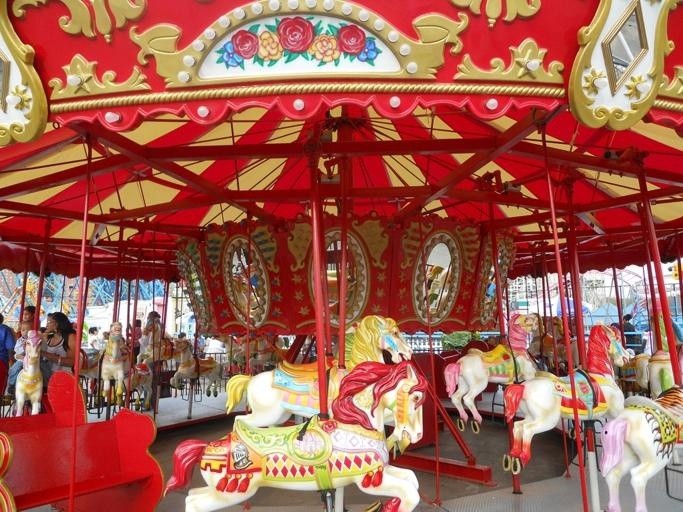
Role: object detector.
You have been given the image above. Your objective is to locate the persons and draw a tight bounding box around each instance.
[0,313,16,368]
[21,306,35,322]
[622,314,636,337]
[87,325,100,349]
[6,321,51,394]
[127,319,142,356]
[248,352,258,375]
[39,312,76,376]
[139,311,161,412]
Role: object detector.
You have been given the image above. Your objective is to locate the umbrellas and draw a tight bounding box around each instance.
[526,295,593,319]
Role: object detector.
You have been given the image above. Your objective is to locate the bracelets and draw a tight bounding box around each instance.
[55,355,62,366]
